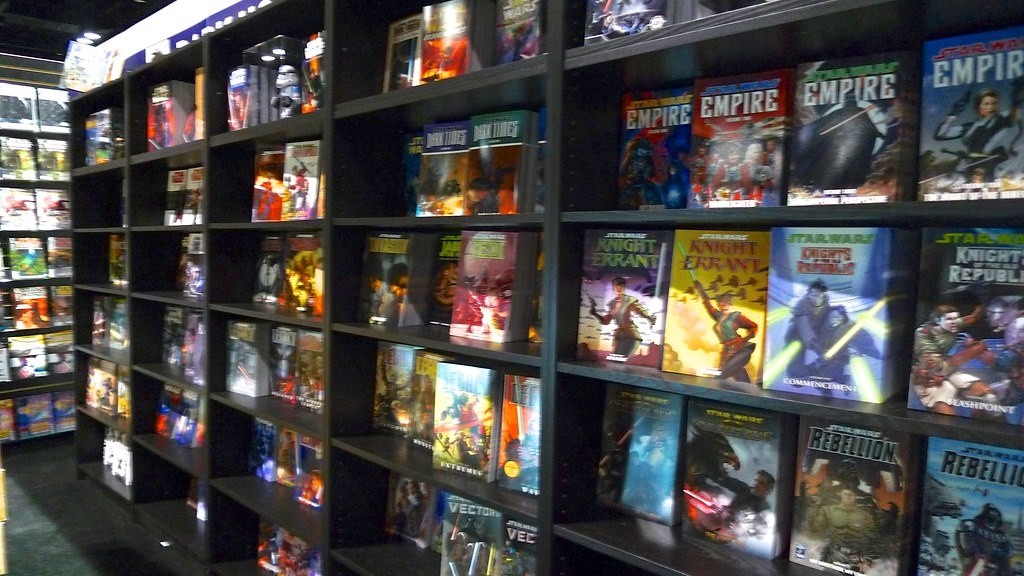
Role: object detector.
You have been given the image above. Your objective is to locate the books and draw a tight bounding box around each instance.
[246,417,323,512]
[58,40,127,93]
[147,66,204,150]
[156,384,203,447]
[369,340,541,496]
[108,234,126,287]
[256,232,324,316]
[382,0,543,94]
[163,167,202,226]
[92,294,129,350]
[177,233,205,300]
[251,139,324,222]
[225,319,324,417]
[590,381,1024,576]
[186,474,209,521]
[257,516,322,576]
[102,425,133,487]
[398,110,546,218]
[86,356,132,418]
[227,28,323,130]
[0,83,79,440]
[163,306,205,385]
[583,0,718,44]
[603,25,1024,211]
[85,107,124,166]
[573,221,1024,427]
[378,474,538,576]
[360,230,542,344]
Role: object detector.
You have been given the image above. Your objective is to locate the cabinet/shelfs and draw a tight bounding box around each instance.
[0,130,75,445]
[68,1,1023,576]
